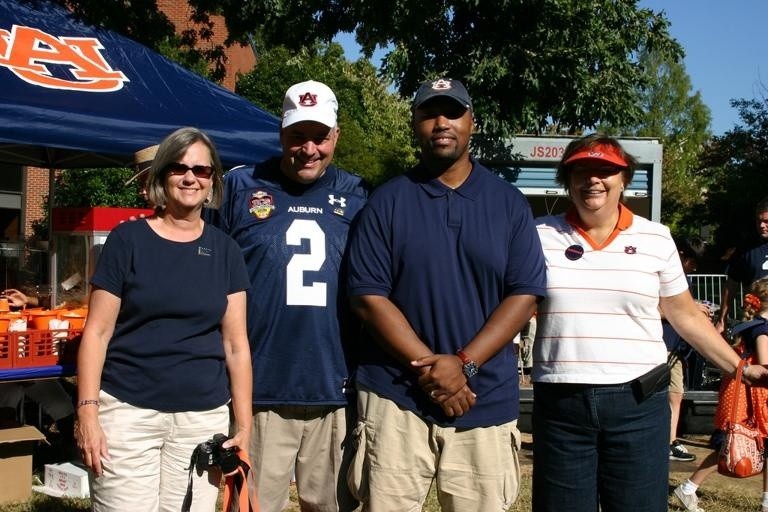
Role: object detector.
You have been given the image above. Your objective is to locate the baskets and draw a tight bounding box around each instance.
[0,327,85,371]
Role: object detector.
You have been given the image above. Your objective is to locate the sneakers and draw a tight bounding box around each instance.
[673,484,706,512]
[668,439,697,463]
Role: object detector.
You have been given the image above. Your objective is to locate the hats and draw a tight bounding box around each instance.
[413,77,474,113]
[281,79,339,129]
[563,142,628,170]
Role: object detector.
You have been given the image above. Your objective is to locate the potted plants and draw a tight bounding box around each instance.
[28,222,49,249]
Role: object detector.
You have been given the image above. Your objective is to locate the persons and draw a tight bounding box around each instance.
[75,126,253,512]
[658,235,711,461]
[350,78,548,512]
[213,79,377,512]
[527,134,768,512]
[1,288,49,309]
[714,200,768,335]
[667,272,768,512]
[123,144,160,207]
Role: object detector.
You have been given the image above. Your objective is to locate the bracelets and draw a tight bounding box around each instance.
[76,400,100,409]
[743,363,750,372]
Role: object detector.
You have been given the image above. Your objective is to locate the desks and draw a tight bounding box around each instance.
[0,365,80,444]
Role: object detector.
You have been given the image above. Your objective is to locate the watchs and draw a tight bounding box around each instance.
[457,351,478,378]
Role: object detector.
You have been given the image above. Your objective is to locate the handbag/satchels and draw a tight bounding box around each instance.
[717,421,766,478]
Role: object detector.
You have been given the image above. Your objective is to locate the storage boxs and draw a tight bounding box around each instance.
[0,425,49,508]
[30,459,95,504]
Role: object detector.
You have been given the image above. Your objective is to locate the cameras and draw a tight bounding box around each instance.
[195,434,241,474]
